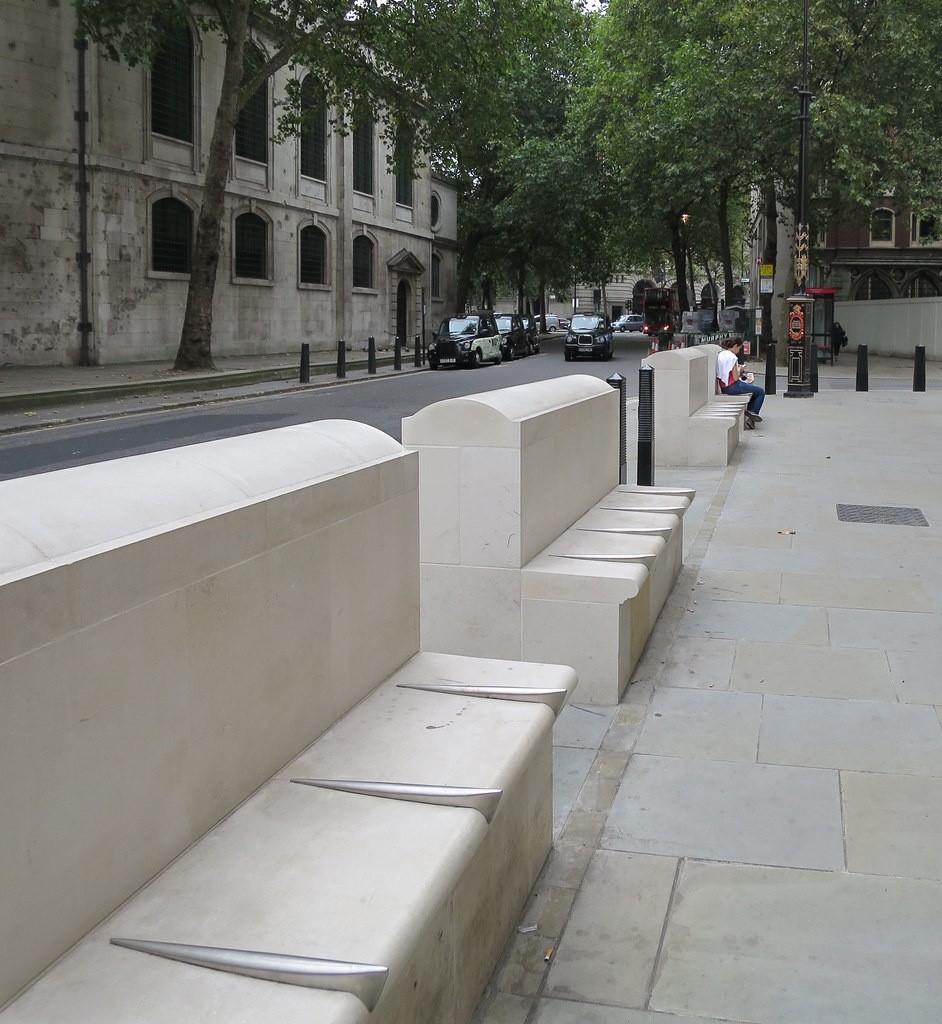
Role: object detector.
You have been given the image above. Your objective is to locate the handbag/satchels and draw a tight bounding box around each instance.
[841,337,848,347]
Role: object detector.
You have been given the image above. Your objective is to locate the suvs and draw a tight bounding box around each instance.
[519,313,541,355]
[561,310,615,361]
[493,312,529,361]
[427,309,503,370]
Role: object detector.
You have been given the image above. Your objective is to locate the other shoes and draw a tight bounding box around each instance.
[835,356,839,361]
[746,418,756,429]
[745,410,762,422]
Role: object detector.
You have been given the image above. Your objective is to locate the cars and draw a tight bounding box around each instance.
[558,317,569,329]
[611,313,644,332]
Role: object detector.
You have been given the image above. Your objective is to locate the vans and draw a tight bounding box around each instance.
[534,313,560,332]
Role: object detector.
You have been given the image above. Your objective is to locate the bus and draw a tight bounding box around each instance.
[643,286,683,336]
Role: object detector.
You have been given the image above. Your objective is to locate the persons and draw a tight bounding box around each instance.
[716,336,765,429]
[833,321,846,361]
[681,318,695,348]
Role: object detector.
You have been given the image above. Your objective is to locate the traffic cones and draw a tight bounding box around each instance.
[649,336,687,355]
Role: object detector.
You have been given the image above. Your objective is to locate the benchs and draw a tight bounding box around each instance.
[0,415,576,1024]
[642,344,750,468]
[400,374,697,705]
